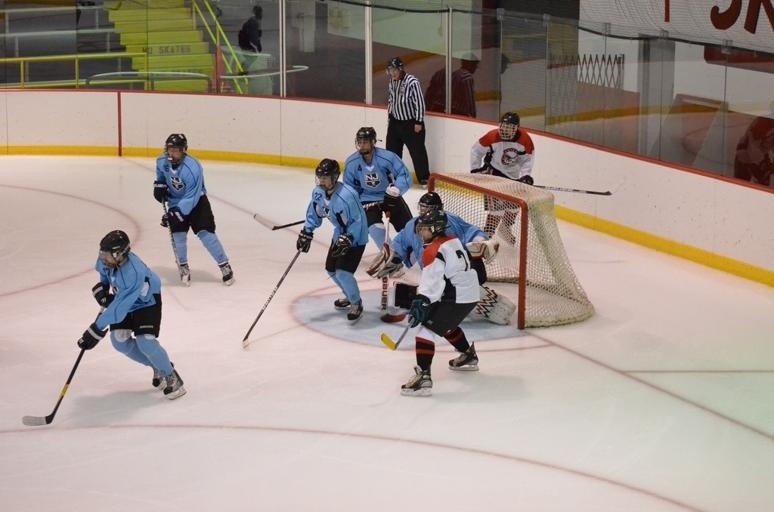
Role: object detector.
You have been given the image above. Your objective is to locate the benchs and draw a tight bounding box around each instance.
[0,0,149,91]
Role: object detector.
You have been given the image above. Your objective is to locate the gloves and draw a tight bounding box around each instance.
[153,180,168,203]
[519,175,533,186]
[77,322,108,349]
[408,295,430,328]
[159,206,183,227]
[383,182,401,218]
[332,233,354,257]
[296,226,313,253]
[92,283,115,308]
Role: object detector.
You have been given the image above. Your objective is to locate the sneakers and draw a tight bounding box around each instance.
[164,369,184,394]
[498,229,517,245]
[152,362,174,387]
[218,261,232,282]
[180,262,190,284]
[335,298,350,307]
[347,298,363,321]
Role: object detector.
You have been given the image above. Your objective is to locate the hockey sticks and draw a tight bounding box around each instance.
[533,180,626,196]
[163,201,190,283]
[381,320,414,349]
[21,306,104,425]
[243,247,302,348]
[381,194,407,323]
[252,203,388,231]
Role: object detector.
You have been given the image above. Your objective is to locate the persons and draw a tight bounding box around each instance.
[342,127,414,251]
[384,58,430,189]
[367,192,516,325]
[401,209,480,390]
[470,112,535,247]
[734,100,774,188]
[297,158,368,320]
[237,4,263,76]
[78,230,183,395]
[153,134,233,282]
[424,52,479,119]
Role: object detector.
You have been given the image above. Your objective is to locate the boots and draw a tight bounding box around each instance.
[449,341,478,367]
[401,365,433,389]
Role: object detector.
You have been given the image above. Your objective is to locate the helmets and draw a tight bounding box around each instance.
[165,133,188,164]
[417,192,444,216]
[416,210,448,245]
[99,230,131,270]
[385,57,404,79]
[497,111,519,141]
[354,127,378,155]
[314,157,341,190]
